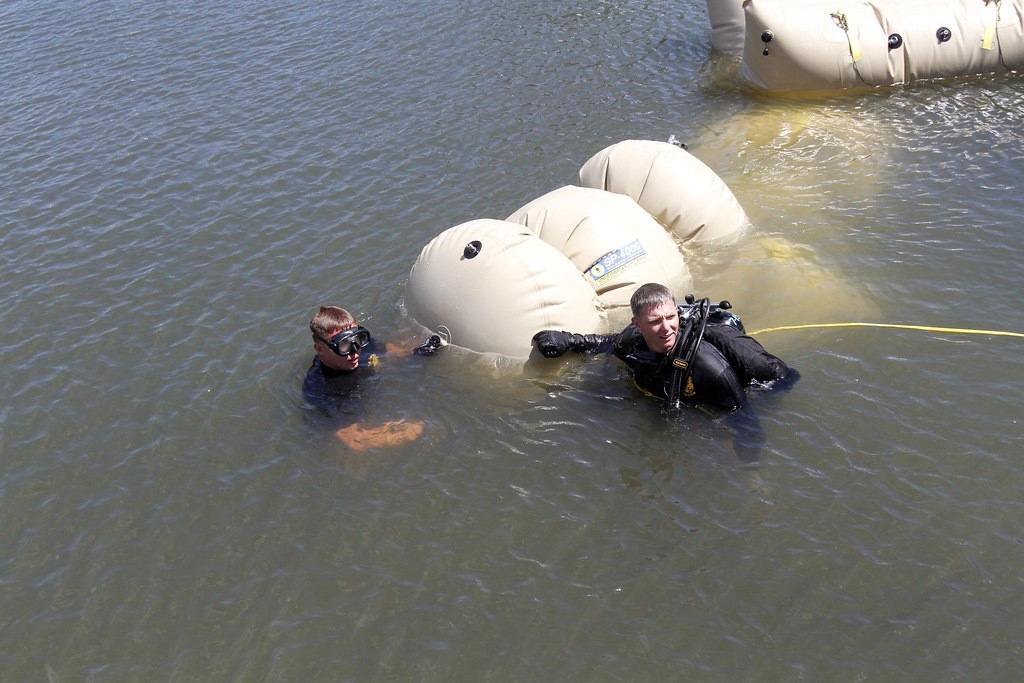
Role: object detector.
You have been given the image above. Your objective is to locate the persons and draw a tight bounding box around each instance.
[307,306,370,385]
[533,282,744,407]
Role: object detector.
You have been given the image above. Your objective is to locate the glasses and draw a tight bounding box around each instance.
[315,325,371,357]
